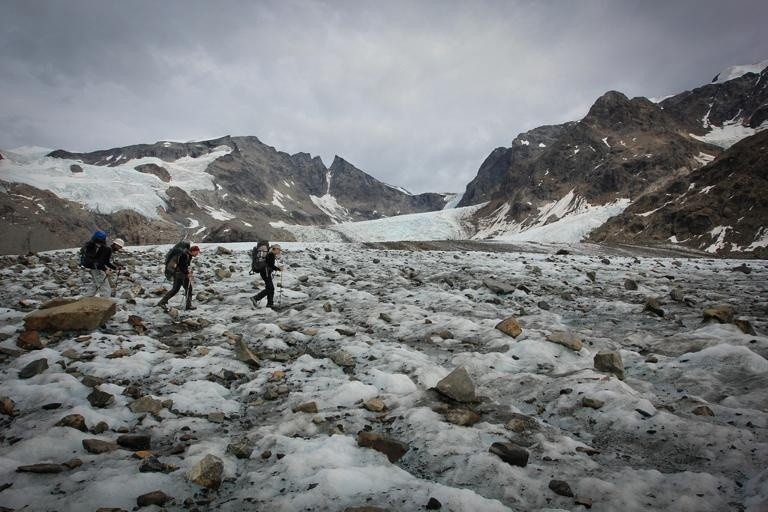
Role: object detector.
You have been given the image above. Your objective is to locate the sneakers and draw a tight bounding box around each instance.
[250,297,258,307]
[266,303,276,308]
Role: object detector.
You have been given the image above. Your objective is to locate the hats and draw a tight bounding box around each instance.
[112,239,124,248]
[272,244,283,251]
[191,246,199,251]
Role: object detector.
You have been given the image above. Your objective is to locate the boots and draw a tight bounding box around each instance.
[157,291,175,310]
[186,294,197,309]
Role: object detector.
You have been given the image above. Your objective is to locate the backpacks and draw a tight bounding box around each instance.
[79,230,113,268]
[165,241,190,279]
[252,241,275,273]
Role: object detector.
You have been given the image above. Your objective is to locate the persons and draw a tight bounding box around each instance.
[157,246,200,311]
[85,238,126,297]
[250,244,282,309]
